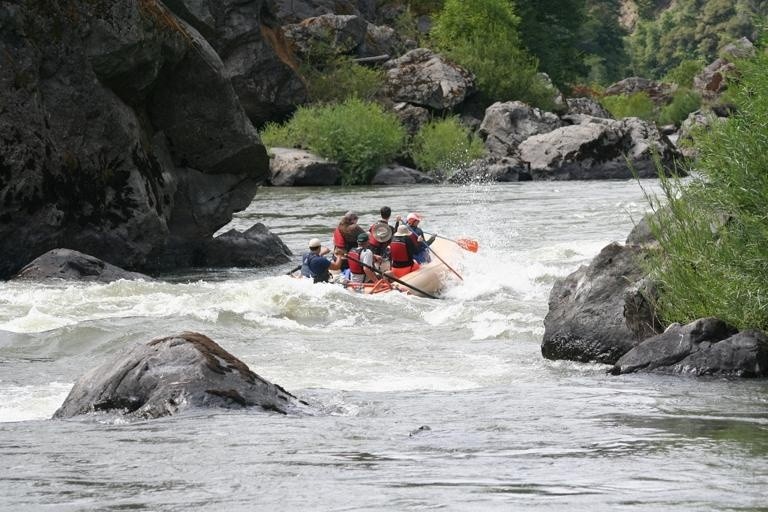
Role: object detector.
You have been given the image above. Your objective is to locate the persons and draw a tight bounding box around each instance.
[332,212,365,272]
[395,212,430,264]
[366,206,395,257]
[347,232,378,292]
[380,225,437,268]
[301,238,344,285]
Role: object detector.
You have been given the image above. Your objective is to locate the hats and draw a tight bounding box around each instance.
[393,224,412,236]
[357,233,369,242]
[309,238,321,248]
[373,223,392,243]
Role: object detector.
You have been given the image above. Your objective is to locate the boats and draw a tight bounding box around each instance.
[329,231,454,297]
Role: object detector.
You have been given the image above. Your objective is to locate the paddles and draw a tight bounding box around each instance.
[399,218,463,280]
[424,231,478,252]
